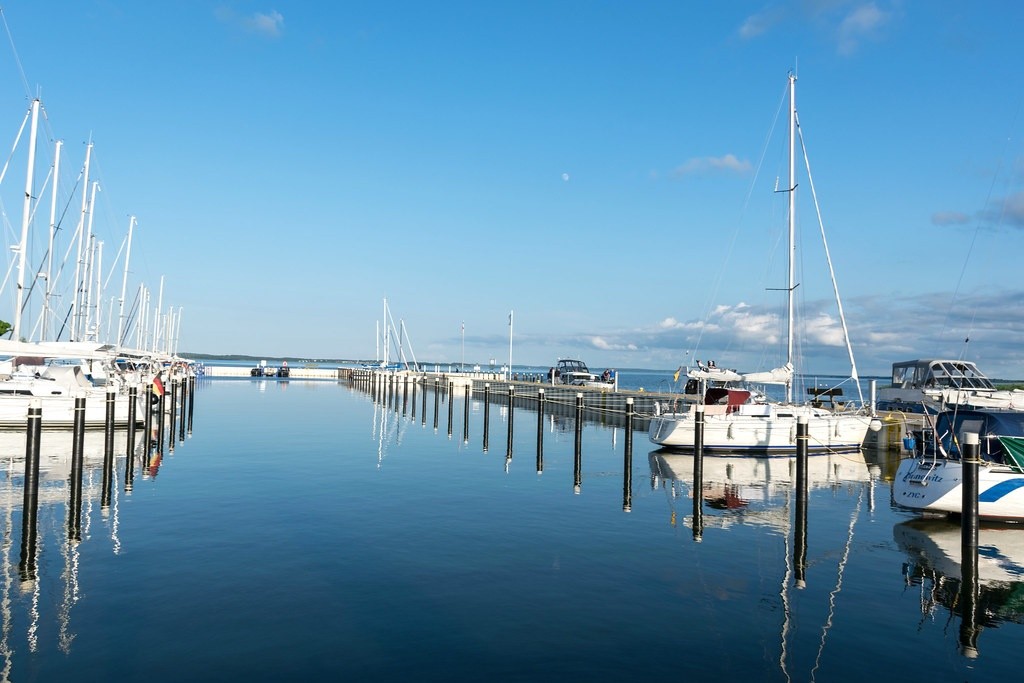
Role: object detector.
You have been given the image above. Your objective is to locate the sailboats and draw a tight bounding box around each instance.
[0,83,198,432]
[351,297,424,384]
[646,447,870,683]
[0,430,146,683]
[649,56,878,455]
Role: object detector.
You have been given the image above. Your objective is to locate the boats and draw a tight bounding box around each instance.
[894,514,1024,640]
[547,357,614,391]
[251,366,289,377]
[893,400,1024,524]
[873,357,1024,419]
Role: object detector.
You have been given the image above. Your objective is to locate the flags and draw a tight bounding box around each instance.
[142,376,164,397]
